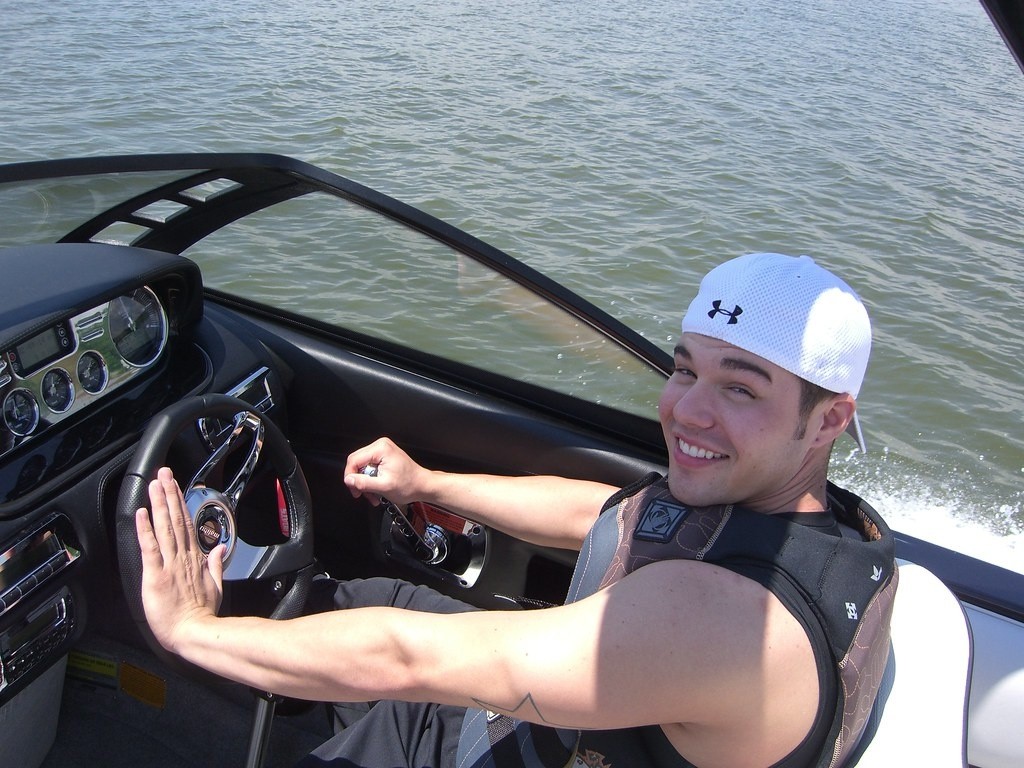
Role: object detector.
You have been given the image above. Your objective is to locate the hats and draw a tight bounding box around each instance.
[682,253,872,454]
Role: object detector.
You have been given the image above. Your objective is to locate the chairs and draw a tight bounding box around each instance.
[847,559,976,768]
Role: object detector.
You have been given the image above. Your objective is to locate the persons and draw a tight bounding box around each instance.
[132,251,900,768]
